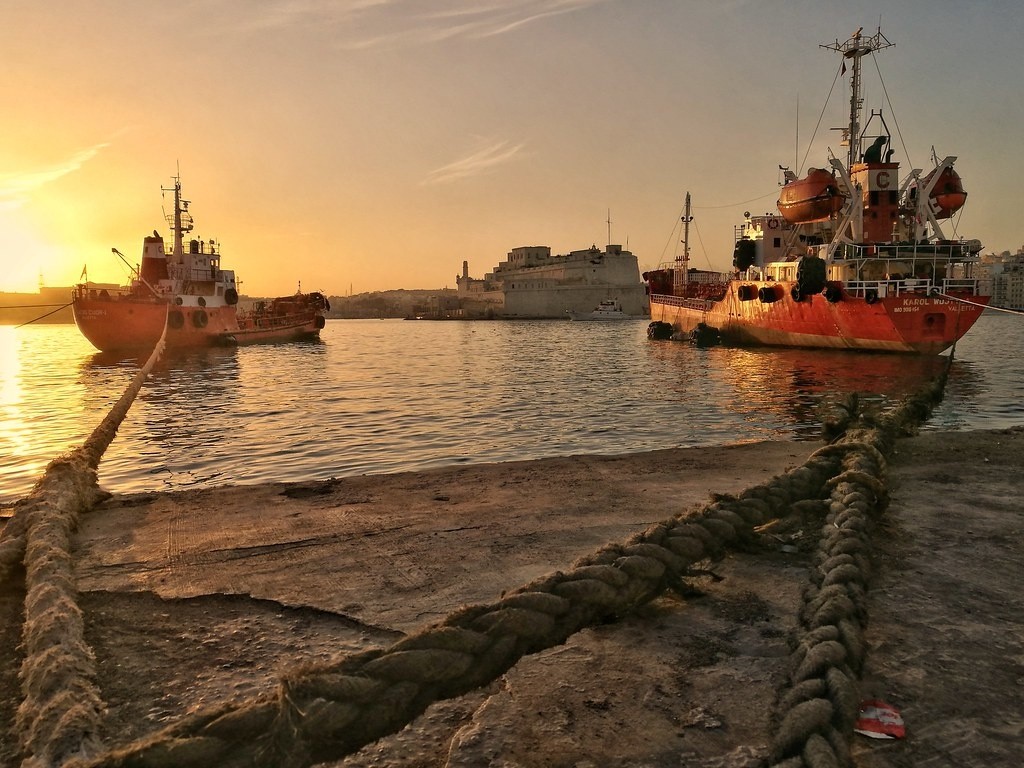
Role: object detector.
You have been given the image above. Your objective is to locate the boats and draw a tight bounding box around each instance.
[564,296,633,321]
[642,18,996,356]
[70,159,331,354]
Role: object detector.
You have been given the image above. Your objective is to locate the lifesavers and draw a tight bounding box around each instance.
[768,218,779,229]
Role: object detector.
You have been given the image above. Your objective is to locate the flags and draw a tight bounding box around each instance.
[916,213,921,224]
[841,61,846,75]
